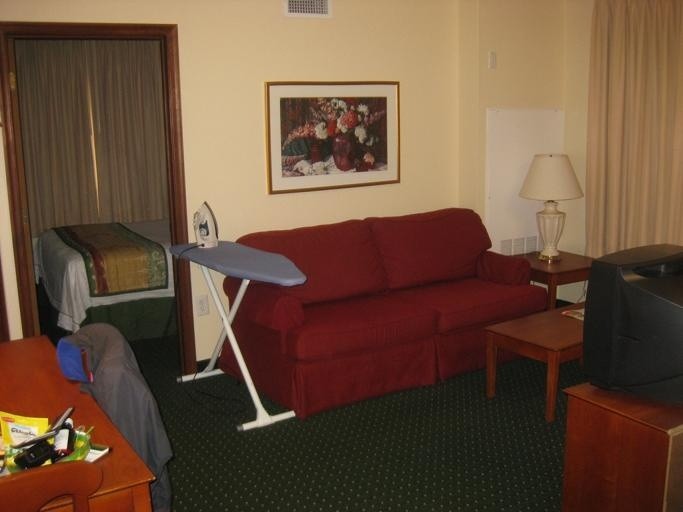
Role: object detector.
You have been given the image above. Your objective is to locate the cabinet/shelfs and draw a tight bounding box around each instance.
[168,238,308,435]
[561,384,683,512]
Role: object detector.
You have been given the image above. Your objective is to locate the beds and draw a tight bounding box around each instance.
[31,219,176,335]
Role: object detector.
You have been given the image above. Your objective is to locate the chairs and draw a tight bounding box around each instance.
[0,323,174,512]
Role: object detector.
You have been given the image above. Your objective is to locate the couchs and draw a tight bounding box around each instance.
[218,207,548,419]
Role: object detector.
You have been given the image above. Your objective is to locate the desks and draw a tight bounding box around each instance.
[484,250,596,423]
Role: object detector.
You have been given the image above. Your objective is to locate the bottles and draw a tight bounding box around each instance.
[53,418,75,458]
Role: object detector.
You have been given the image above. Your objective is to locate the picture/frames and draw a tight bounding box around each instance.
[265,80,400,195]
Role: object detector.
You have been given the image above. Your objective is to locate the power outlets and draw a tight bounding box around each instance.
[195,294,209,316]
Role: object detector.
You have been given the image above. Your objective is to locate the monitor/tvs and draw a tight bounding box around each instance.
[582,244,683,405]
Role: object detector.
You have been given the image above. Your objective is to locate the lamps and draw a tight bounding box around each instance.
[519,154,585,264]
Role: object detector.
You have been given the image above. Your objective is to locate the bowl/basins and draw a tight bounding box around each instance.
[5,433,90,473]
